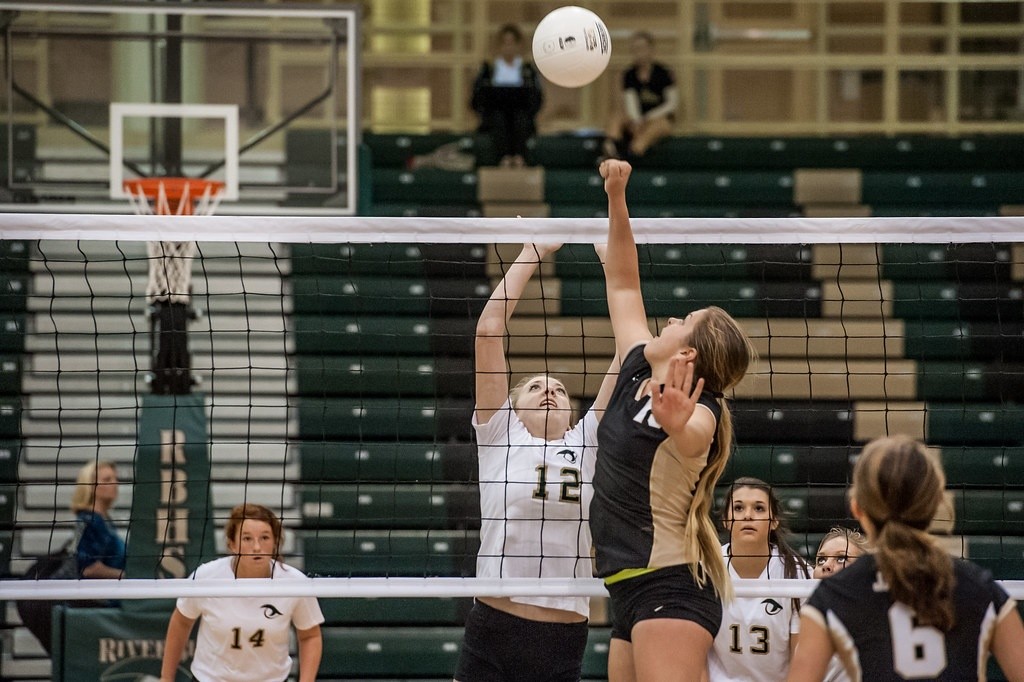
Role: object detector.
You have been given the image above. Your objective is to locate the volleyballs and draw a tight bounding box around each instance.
[531,5,613,88]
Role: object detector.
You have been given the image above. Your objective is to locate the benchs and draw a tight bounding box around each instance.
[0,119,1024,682]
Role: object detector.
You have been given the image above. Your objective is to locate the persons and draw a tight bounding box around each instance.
[710,479,813,682]
[471,24,546,168]
[604,29,678,171]
[787,437,1024,682]
[162,504,325,682]
[590,160,752,682]
[74,462,127,578]
[454,243,621,682]
